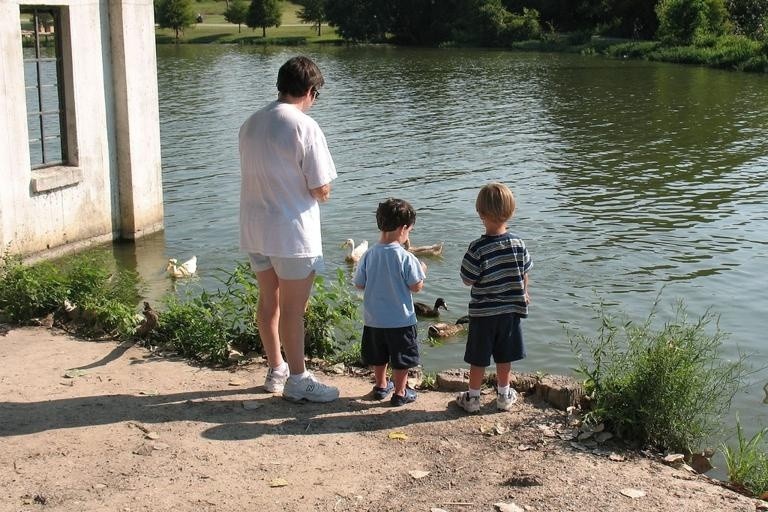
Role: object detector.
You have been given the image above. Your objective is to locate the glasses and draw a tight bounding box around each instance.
[312,85,319,99]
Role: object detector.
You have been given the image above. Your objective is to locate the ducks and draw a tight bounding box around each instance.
[341,238,368,262]
[414,298,448,318]
[134,302,159,339]
[403,238,443,256]
[428,315,470,338]
[165,256,197,278]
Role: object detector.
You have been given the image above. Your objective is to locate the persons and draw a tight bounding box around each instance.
[352,197,429,406]
[235,55,342,405]
[452,181,534,415]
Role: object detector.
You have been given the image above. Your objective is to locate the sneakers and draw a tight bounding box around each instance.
[497,388,518,411]
[264,363,291,393]
[283,373,338,403]
[374,381,394,400]
[391,390,416,406]
[456,393,479,414]
